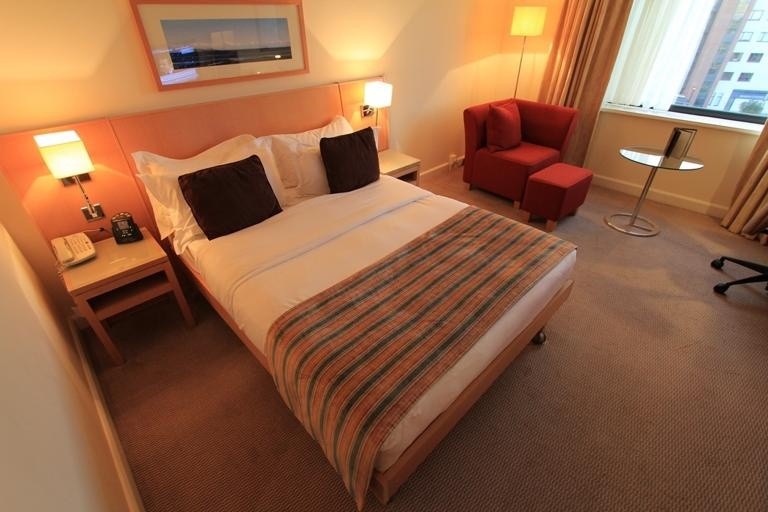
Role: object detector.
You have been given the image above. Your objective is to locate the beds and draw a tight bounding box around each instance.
[110,82,578,512]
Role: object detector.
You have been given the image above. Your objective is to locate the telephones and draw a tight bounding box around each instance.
[51,232,96,268]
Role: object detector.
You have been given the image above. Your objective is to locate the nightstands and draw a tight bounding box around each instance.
[61,227,194,365]
[375,150,421,185]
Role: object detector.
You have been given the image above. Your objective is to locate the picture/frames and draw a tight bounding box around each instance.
[129,1,311,92]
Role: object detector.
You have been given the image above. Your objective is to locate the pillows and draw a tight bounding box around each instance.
[178,154,283,241]
[287,128,379,197]
[487,102,521,153]
[130,133,257,241]
[271,115,354,187]
[137,137,288,256]
[319,126,380,194]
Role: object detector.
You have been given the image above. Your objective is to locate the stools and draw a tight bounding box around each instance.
[520,163,592,231]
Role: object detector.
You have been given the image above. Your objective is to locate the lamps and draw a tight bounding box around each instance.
[359,81,392,117]
[35,130,105,222]
[510,4,547,98]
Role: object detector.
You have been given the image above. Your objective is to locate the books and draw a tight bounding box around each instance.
[664,126,697,160]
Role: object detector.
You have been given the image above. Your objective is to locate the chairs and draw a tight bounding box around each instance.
[463,97,581,209]
